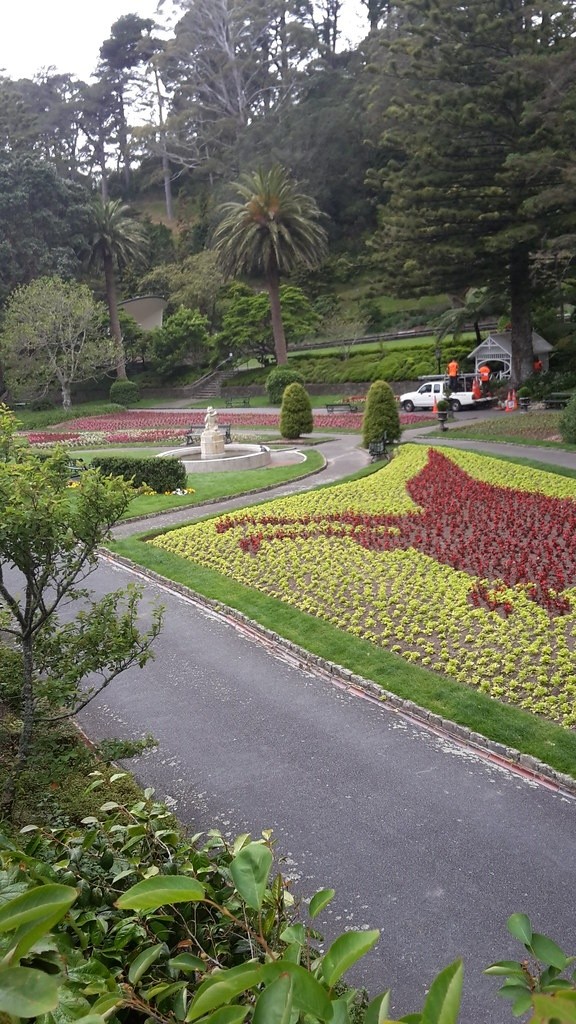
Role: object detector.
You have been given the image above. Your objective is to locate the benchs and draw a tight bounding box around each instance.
[226,395,251,408]
[187,424,233,444]
[325,403,358,413]
[544,393,571,409]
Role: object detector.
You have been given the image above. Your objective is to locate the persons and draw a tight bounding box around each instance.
[477,364,491,398]
[534,356,542,372]
[448,357,459,393]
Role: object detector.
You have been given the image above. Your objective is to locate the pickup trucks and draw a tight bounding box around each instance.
[399,381,498,412]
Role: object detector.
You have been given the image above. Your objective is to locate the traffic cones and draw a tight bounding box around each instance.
[512,388,518,409]
[472,379,481,399]
[431,395,438,414]
[505,392,513,413]
